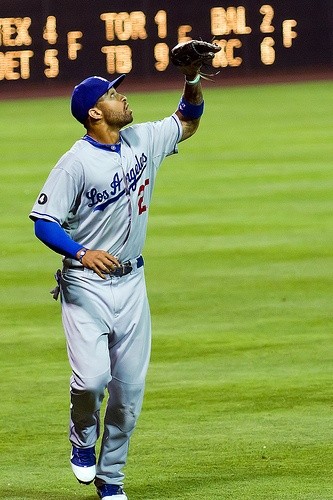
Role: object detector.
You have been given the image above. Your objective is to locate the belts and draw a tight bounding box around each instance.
[69,256,143,275]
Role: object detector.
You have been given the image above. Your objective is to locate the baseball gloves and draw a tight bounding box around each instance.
[169,39,222,83]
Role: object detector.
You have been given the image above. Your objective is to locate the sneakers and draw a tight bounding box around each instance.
[70,445,97,482]
[98,484,127,500]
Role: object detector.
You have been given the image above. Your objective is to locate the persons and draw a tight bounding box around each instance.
[29,40,221,500]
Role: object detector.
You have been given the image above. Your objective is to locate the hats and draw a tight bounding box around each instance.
[70,74,128,124]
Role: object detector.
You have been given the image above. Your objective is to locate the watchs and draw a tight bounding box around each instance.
[79,248,89,263]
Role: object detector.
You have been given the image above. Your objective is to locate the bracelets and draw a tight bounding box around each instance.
[184,73,200,85]
